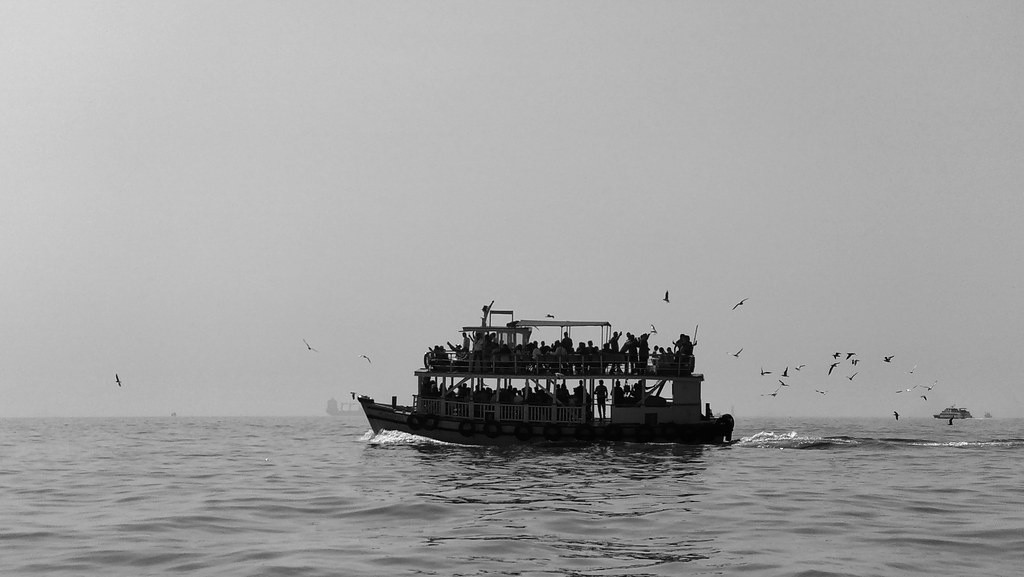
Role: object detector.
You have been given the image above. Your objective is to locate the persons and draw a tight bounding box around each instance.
[609,330,624,375]
[422,376,592,422]
[594,380,609,422]
[428,328,611,374]
[628,379,657,406]
[610,380,628,406]
[620,331,698,375]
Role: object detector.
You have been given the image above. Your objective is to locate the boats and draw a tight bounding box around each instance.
[934,405,974,419]
[356,300,742,445]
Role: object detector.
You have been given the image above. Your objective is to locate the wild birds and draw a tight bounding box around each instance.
[781,366,790,377]
[116,373,121,387]
[846,352,860,380]
[795,365,806,370]
[350,392,356,400]
[761,368,772,375]
[895,363,937,401]
[732,348,744,358]
[359,355,371,363]
[893,411,899,420]
[731,297,748,310]
[651,324,657,334]
[947,415,955,425]
[662,290,670,303]
[828,362,839,375]
[303,339,317,352]
[884,355,895,362]
[768,380,790,398]
[545,314,555,318]
[832,352,841,359]
